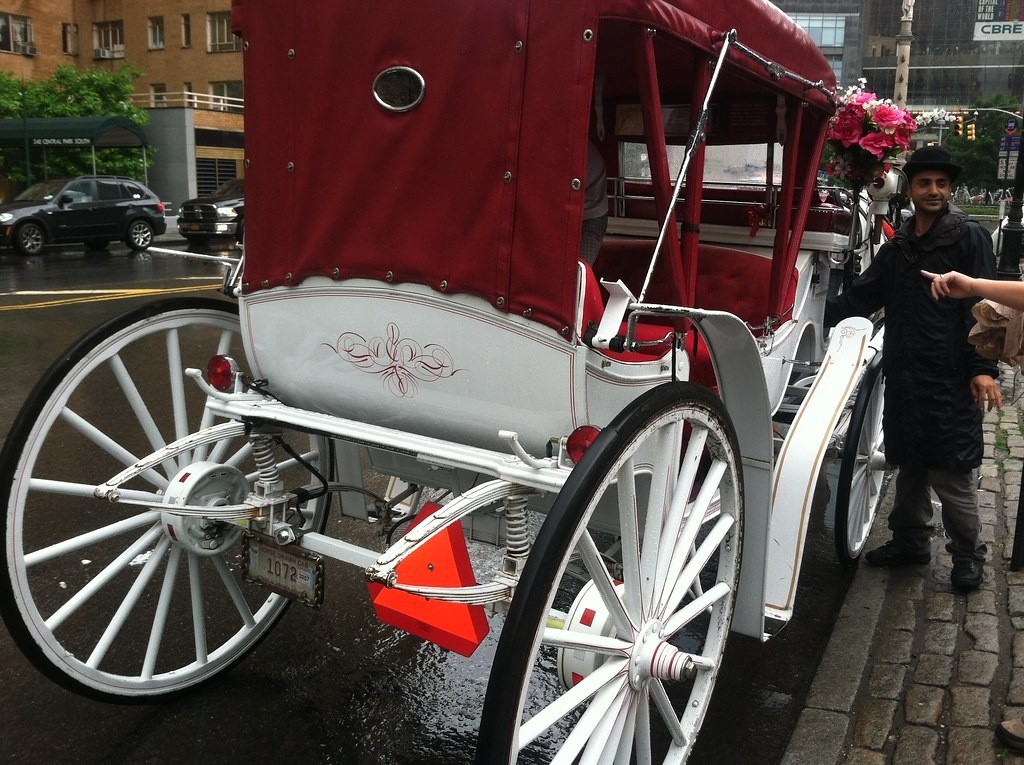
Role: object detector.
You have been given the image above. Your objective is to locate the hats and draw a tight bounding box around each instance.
[900,146,963,185]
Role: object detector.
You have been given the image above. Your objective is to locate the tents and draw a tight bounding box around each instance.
[0,117,149,187]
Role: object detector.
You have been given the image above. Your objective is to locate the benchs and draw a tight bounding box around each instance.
[577,239,799,390]
[622,181,853,236]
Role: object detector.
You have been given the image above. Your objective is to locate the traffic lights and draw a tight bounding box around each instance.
[966,123,976,142]
[954,116,963,138]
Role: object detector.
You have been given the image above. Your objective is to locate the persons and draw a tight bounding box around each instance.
[579,136,609,268]
[824,145,1024,750]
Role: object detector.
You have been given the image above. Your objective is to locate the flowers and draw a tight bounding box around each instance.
[824,76,956,204]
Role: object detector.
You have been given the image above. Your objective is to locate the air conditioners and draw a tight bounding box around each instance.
[21,45,37,56]
[95,49,110,58]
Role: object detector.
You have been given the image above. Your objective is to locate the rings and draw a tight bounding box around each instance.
[940,273,945,279]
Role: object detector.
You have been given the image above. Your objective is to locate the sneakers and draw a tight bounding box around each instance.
[950,559,983,587]
[865,537,932,565]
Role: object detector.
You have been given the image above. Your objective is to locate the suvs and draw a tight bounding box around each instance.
[0,175,167,256]
[178,178,245,243]
